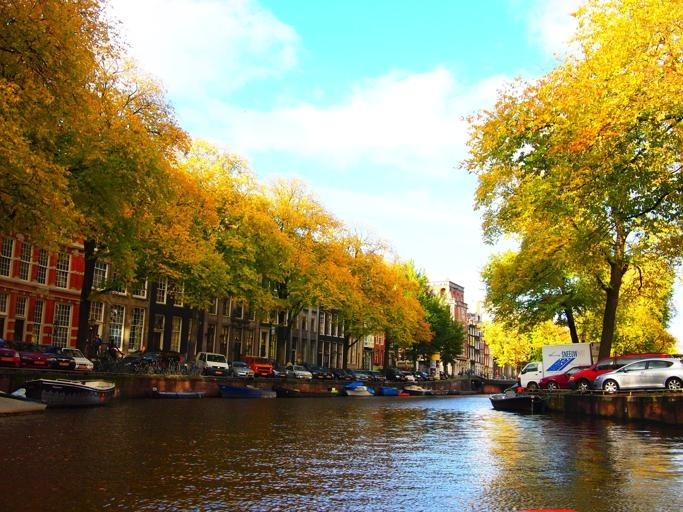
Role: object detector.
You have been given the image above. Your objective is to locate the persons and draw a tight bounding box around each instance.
[110,346,117,360]
[95,335,101,354]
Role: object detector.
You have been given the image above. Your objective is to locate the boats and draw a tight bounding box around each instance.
[278,380,459,397]
[24,378,117,405]
[214,381,276,399]
[0,390,47,414]
[488,393,544,414]
[148,383,208,398]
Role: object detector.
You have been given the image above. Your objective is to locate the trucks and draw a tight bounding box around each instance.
[515,340,592,393]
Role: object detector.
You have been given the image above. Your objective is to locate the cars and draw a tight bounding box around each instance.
[0,337,274,379]
[592,356,683,393]
[274,364,447,384]
[538,364,592,392]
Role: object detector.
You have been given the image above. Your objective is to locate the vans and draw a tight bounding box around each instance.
[568,354,670,390]
